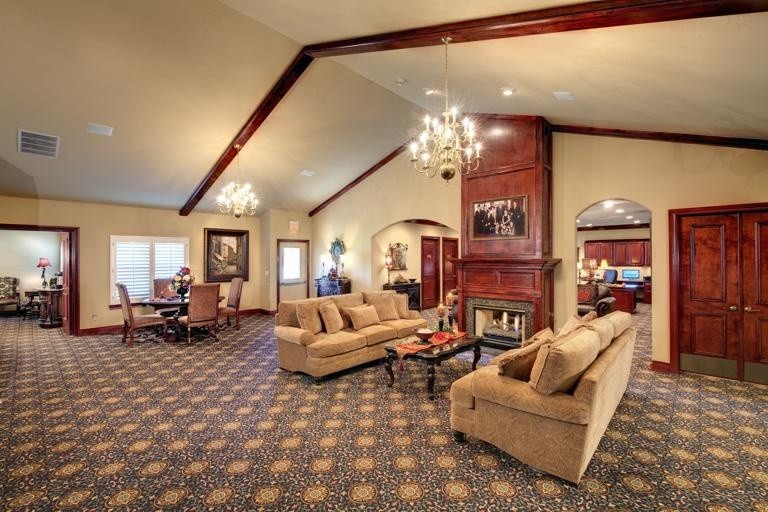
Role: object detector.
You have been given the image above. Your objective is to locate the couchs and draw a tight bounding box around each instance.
[578,280,616,316]
[446,309,636,486]
[272,289,427,386]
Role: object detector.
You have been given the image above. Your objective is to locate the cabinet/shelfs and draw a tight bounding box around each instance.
[383,281,421,312]
[644,283,651,304]
[585,241,613,265]
[315,277,351,298]
[613,241,644,265]
[644,240,650,265]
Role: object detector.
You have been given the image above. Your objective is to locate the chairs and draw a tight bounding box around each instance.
[22,291,40,321]
[218,278,244,330]
[115,282,167,348]
[0,276,21,317]
[175,284,222,346]
[153,278,180,338]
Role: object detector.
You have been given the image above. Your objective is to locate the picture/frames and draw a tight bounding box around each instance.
[470,194,530,240]
[204,228,250,283]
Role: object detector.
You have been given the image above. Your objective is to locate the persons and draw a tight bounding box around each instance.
[474,201,521,236]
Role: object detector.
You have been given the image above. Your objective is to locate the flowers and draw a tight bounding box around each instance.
[168,266,196,289]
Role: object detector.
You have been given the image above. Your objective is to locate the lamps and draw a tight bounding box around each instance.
[386,252,392,285]
[319,254,327,278]
[216,143,259,220]
[36,257,52,288]
[407,32,484,184]
[338,254,346,278]
[582,258,598,282]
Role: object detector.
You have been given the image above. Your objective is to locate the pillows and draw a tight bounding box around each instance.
[346,305,381,330]
[294,303,323,335]
[319,299,345,337]
[360,290,400,322]
[496,310,600,379]
[339,301,369,332]
[391,294,409,319]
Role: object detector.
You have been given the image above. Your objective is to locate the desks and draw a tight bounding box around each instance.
[37,289,63,328]
[578,281,638,315]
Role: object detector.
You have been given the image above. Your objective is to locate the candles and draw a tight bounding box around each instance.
[437,303,445,318]
[446,292,454,306]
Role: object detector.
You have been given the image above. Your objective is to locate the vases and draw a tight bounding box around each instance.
[176,285,188,299]
